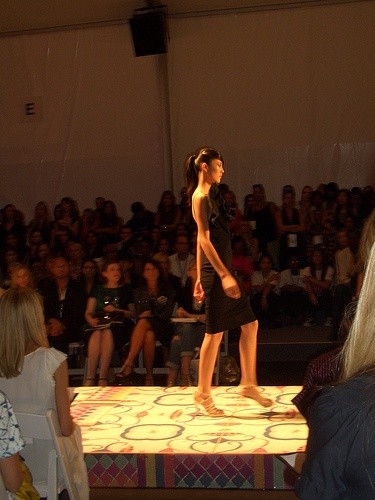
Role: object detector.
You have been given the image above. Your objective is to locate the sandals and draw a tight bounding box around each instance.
[85,378,109,386]
[237,384,272,407]
[193,390,224,415]
[114,364,136,379]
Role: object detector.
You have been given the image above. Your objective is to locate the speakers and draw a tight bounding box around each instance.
[129,11,168,57]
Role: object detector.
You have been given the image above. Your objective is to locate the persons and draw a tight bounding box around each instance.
[290,208,375,500]
[0,182,375,388]
[186,148,272,416]
[0,388,40,500]
[0,287,84,500]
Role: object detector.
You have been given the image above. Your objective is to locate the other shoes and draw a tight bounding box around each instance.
[324,317,335,326]
[167,374,193,387]
[304,318,317,327]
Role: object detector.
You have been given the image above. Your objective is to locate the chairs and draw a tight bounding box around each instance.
[15,409,80,500]
[69,330,229,386]
